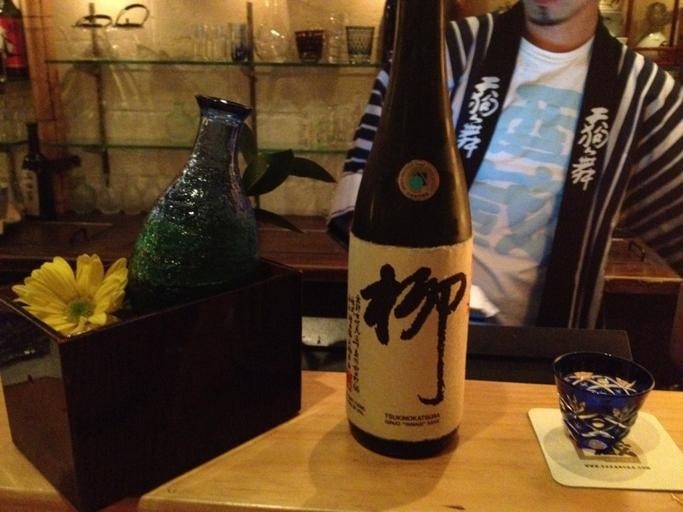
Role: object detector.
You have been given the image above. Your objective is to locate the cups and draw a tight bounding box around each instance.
[347,26,376,63]
[552,350,658,452]
[295,28,324,64]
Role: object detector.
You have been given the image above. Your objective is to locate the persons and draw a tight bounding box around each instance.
[326,2,682,331]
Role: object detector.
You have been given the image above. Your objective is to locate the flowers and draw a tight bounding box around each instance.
[14,252,131,337]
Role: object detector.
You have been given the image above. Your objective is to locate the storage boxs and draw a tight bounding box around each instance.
[1,258,301,512]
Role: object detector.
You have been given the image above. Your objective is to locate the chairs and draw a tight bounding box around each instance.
[466,324,635,383]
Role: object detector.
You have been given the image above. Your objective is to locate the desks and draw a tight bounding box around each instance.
[1,372,683,511]
[2,213,683,374]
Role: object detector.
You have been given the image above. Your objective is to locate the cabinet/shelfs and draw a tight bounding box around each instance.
[41,59,379,157]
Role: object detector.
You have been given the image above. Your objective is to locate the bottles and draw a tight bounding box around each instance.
[19,120,50,220]
[123,93,262,312]
[345,0,475,459]
[190,20,247,63]
[1,0,30,82]
